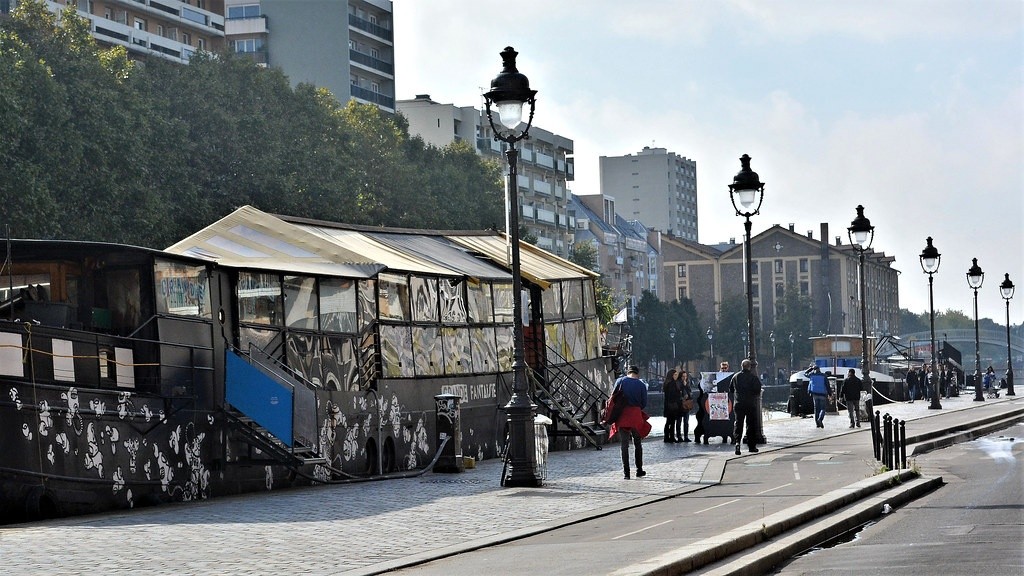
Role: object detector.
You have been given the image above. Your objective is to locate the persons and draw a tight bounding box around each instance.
[729,359,761,456]
[719,361,730,372]
[804,365,833,429]
[918,363,952,401]
[662,370,681,443]
[676,371,691,442]
[906,365,918,404]
[841,369,862,428]
[986,366,995,388]
[613,365,647,479]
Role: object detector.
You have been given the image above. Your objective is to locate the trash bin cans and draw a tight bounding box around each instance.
[790,381,808,416]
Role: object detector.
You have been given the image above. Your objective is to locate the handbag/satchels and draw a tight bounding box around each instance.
[682,400,693,409]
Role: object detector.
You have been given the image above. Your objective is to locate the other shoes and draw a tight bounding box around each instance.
[735,445,741,455]
[664,439,673,443]
[624,476,630,480]
[636,471,646,477]
[678,437,683,442]
[749,448,759,452]
[857,424,861,427]
[817,419,824,428]
[669,437,679,443]
[849,424,855,428]
[684,438,691,442]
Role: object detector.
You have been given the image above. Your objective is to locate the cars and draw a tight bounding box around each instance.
[639,379,649,391]
[649,380,663,391]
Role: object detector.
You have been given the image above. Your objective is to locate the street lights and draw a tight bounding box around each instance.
[999,273,1016,395]
[707,326,715,371]
[966,258,985,402]
[789,331,795,373]
[482,47,544,487]
[669,325,676,368]
[769,330,779,385]
[728,154,765,445]
[847,204,875,421]
[919,236,942,409]
[740,329,747,358]
